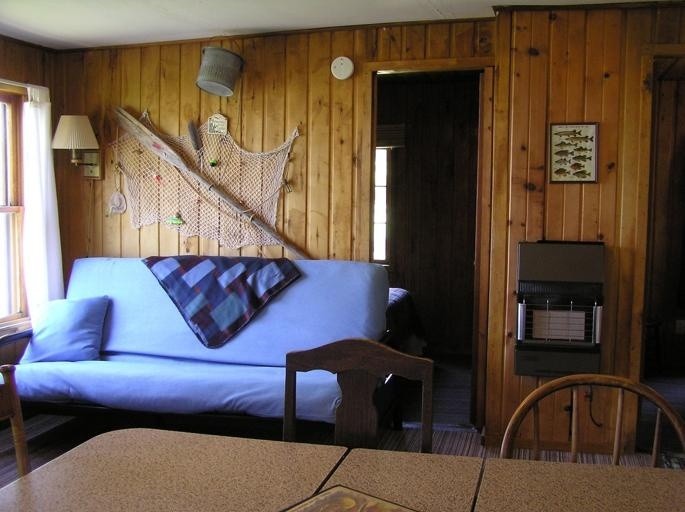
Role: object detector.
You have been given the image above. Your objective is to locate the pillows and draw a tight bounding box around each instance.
[19,295,108,364]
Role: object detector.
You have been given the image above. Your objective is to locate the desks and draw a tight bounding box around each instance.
[0,426,685,511]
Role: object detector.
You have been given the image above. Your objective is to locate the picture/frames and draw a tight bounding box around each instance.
[550,122,599,184]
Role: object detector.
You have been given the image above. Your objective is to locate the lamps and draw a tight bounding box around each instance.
[50,115,103,180]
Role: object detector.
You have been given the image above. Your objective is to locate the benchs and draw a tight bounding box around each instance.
[0,257,389,430]
